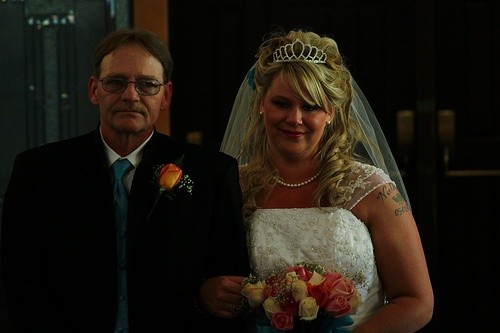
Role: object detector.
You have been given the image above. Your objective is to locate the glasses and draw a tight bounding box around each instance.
[96,76,164,96]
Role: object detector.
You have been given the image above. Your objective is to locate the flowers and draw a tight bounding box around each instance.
[150,164,194,201]
[229,261,369,333]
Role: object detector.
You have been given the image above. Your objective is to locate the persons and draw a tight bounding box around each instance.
[0,29,250,333]
[200,30,434,333]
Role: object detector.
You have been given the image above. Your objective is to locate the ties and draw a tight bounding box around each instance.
[110,159,135,333]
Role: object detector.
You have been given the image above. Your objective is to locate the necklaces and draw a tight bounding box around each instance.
[272,168,322,187]
